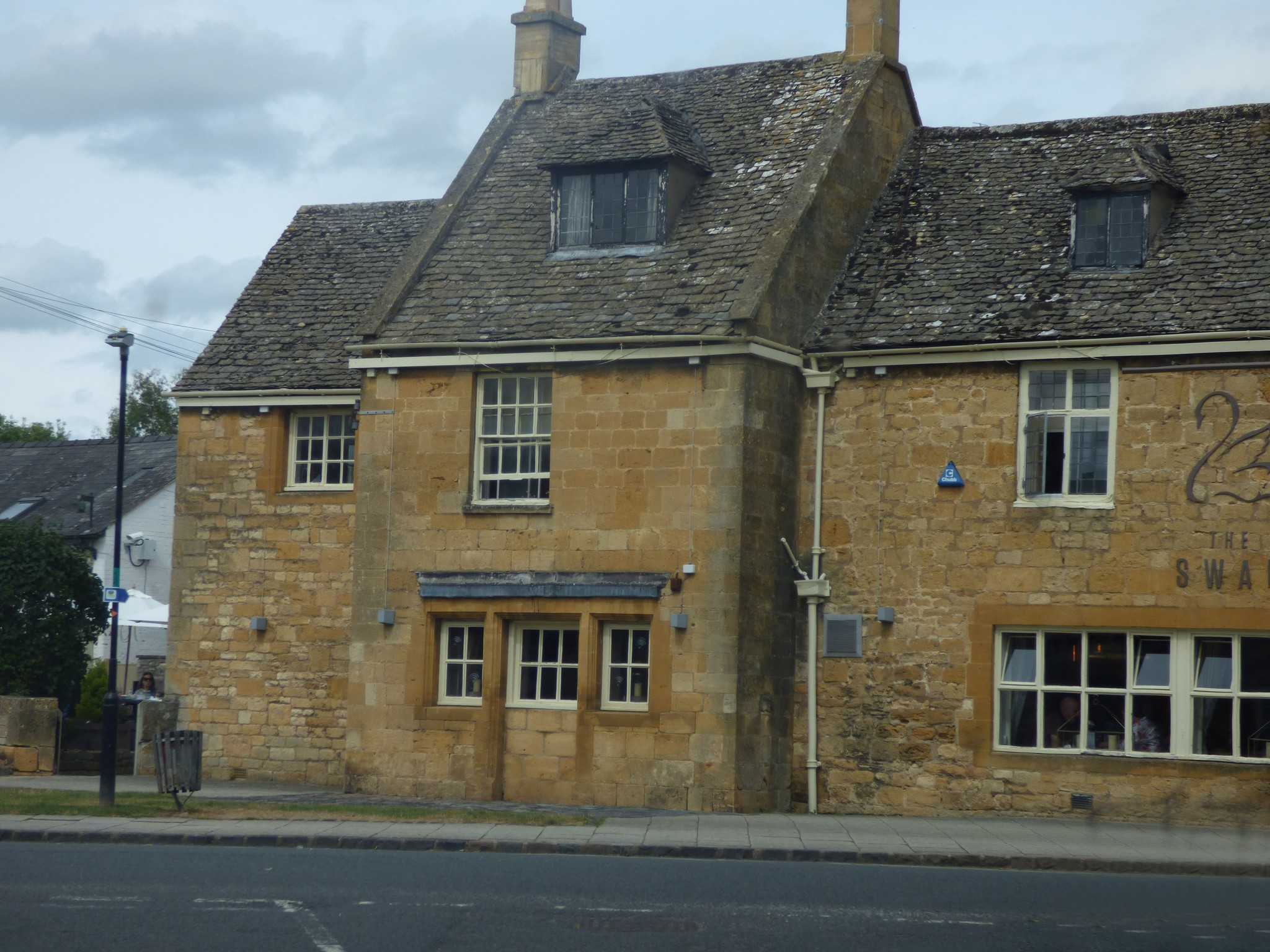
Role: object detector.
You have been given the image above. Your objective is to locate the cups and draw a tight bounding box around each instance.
[1265,742,1270,757]
[1108,735,1118,749]
[473,678,481,692]
[1076,735,1080,748]
[634,683,641,696]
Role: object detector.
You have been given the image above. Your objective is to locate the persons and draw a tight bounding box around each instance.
[1045,696,1109,749]
[134,671,155,700]
[1113,706,1162,752]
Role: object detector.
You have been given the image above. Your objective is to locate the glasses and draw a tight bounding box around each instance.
[143,678,153,682]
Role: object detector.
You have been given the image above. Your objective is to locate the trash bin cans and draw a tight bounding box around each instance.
[153,729,202,792]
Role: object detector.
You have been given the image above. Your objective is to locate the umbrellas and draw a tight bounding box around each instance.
[129,603,168,624]
[84,586,168,696]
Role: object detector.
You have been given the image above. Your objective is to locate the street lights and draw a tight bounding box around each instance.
[99,327,134,808]
